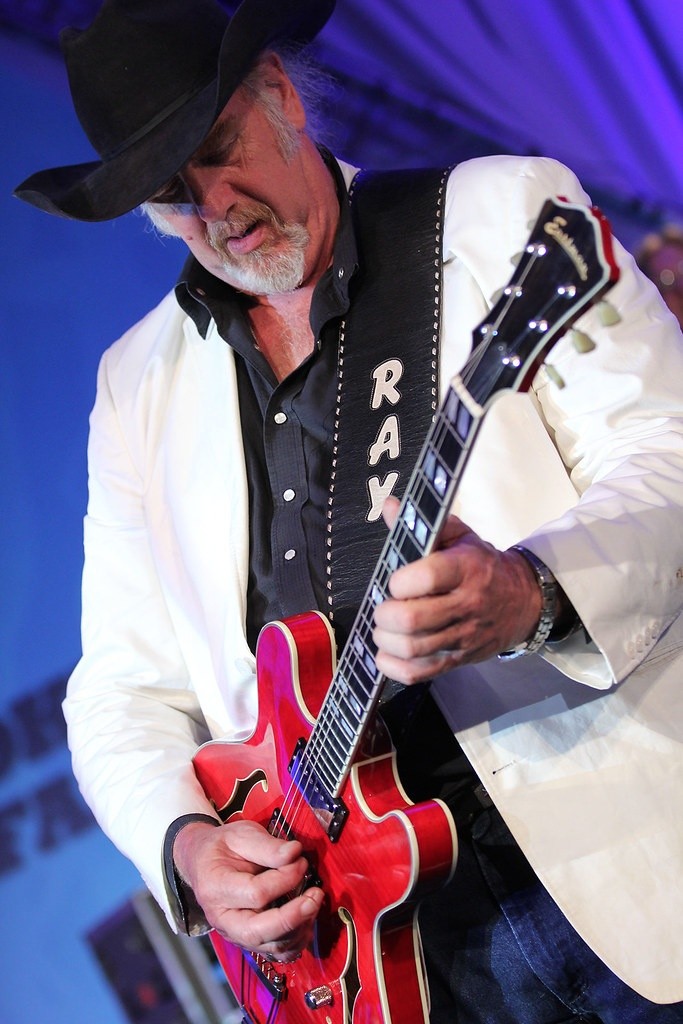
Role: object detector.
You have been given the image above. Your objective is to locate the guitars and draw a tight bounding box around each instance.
[187,190,627,1024]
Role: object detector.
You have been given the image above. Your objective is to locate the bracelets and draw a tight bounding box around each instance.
[495,543,560,662]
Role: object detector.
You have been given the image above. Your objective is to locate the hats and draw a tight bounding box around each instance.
[11,0,338,221]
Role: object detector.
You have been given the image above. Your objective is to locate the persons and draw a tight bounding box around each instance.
[16,1,682,1024]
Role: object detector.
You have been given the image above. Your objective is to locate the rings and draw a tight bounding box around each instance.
[266,951,274,963]
